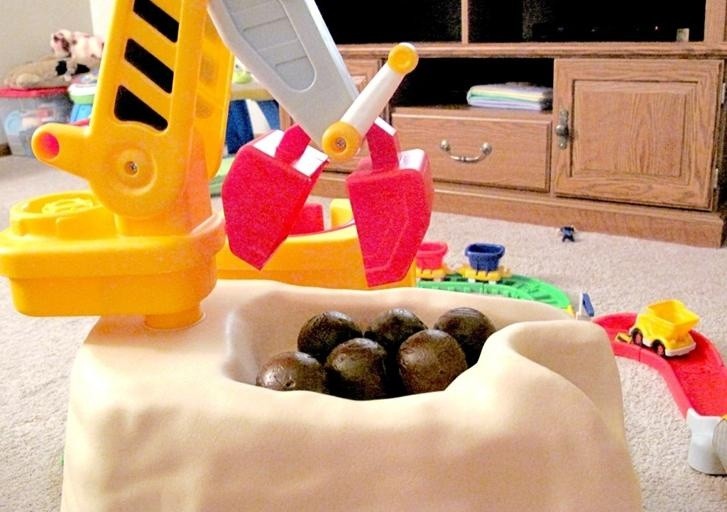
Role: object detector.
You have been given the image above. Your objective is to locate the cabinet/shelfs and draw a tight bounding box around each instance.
[280,0,727,246]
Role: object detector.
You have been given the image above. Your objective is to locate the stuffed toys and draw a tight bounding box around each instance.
[3,55,73,93]
[48,28,105,77]
